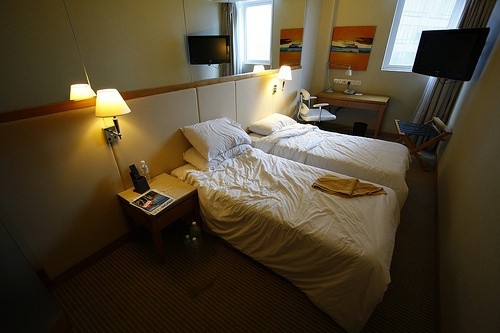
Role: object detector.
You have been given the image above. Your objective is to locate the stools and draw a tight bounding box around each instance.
[394,116,453,162]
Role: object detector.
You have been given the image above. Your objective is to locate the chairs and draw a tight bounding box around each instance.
[297,88,337,127]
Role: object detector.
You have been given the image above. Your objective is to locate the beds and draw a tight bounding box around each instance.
[248,123,412,207]
[172,148,400,333]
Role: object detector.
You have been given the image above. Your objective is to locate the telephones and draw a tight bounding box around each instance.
[344,88,356,95]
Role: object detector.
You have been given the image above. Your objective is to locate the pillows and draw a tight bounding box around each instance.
[248,113,298,136]
[179,118,252,162]
[182,144,252,171]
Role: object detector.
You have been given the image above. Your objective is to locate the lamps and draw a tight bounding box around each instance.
[325,62,352,92]
[95,88,131,146]
[70,83,96,101]
[273,65,292,92]
[252,64,266,73]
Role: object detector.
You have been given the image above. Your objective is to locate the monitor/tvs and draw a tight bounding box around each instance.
[411,28,491,82]
[187,35,231,65]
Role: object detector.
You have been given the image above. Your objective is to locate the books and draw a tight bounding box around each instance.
[129,190,177,216]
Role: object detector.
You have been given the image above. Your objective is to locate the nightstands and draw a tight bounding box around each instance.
[117,171,203,264]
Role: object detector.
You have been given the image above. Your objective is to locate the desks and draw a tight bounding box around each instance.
[316,91,390,138]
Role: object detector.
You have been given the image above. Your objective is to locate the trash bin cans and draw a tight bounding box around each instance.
[353,122,368,136]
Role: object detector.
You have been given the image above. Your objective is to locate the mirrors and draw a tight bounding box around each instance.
[0,0,309,124]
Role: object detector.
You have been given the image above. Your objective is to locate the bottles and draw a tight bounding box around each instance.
[345,67,352,80]
[140,161,151,186]
